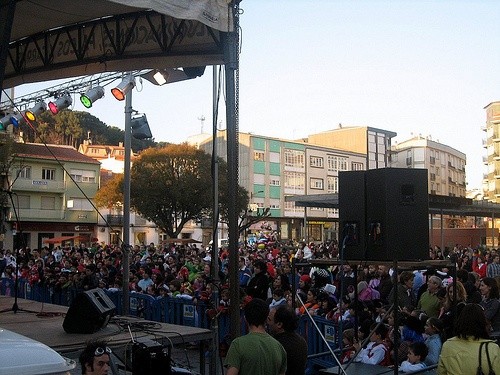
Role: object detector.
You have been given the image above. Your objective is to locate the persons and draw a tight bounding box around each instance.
[268,304,308,375]
[0,234,500,375]
[78,341,112,375]
[224,297,287,375]
[436,302,500,375]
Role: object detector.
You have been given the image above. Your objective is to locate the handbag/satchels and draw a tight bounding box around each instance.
[475,342,496,375]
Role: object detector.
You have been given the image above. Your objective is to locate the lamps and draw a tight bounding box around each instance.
[24,96,47,122]
[80,80,105,108]
[0,106,18,130]
[47,89,73,116]
[131,112,153,140]
[110,70,139,102]
[10,104,30,127]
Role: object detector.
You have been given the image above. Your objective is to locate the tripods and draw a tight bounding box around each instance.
[0,194,39,313]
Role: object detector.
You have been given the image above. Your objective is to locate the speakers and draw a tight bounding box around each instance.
[63,287,117,334]
[337,168,429,261]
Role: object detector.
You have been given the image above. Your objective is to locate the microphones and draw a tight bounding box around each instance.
[0,187,16,194]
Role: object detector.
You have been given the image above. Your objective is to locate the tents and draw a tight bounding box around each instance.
[43,234,98,242]
[162,238,202,243]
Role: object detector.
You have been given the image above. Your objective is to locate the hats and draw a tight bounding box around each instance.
[300,275,310,282]
